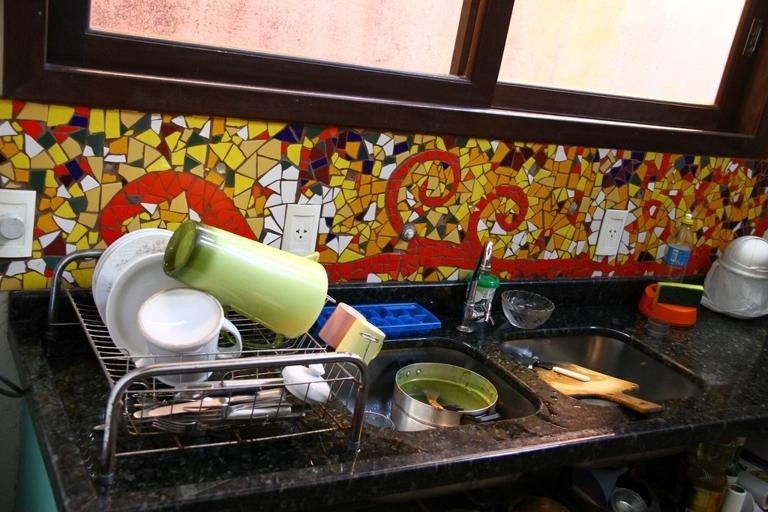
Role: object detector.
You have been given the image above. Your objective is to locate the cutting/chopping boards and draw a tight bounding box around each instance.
[537,363,663,414]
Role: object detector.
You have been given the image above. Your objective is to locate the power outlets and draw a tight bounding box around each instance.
[283,202,321,255]
[594,209,628,256]
[0,188,37,258]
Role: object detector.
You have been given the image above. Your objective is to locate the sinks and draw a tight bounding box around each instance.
[295,339,542,429]
[498,325,706,407]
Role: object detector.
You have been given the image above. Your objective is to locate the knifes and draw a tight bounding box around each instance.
[530,359,591,382]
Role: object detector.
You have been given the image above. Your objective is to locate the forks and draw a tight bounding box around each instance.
[130,388,295,437]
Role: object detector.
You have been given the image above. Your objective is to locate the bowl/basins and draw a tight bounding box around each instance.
[501,289,554,330]
[509,486,648,511]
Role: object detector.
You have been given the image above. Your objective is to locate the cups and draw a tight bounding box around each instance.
[317,302,367,350]
[464,272,499,323]
[141,287,243,385]
[335,318,386,367]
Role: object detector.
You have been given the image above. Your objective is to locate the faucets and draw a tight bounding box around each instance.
[461,241,494,326]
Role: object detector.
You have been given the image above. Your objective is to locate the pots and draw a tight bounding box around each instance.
[388,359,500,433]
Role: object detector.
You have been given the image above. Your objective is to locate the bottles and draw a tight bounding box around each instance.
[661,212,694,282]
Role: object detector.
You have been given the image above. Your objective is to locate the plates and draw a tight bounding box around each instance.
[93,228,187,358]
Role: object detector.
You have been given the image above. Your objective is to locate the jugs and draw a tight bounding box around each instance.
[161,217,329,349]
